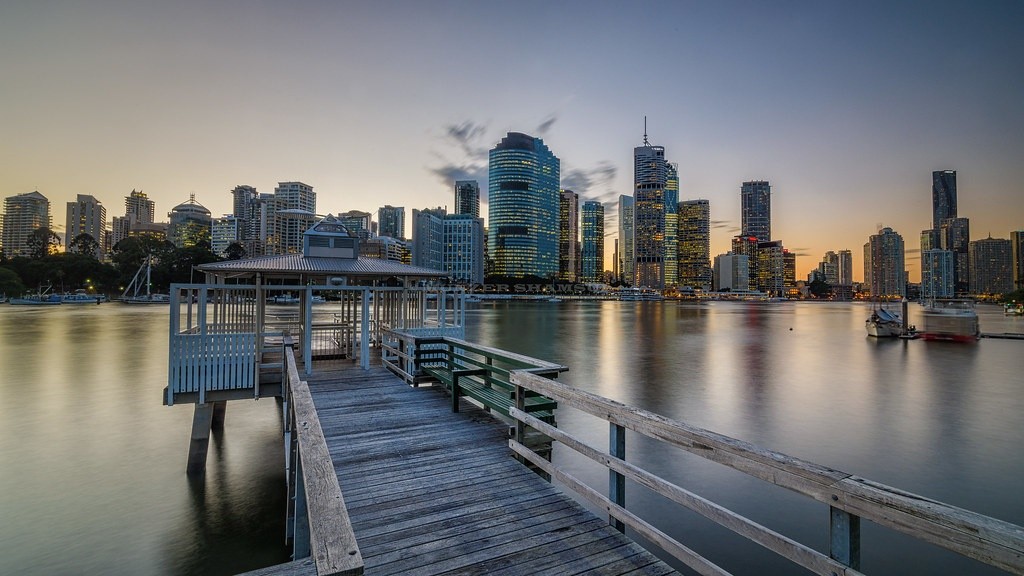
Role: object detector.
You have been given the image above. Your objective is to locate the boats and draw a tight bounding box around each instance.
[865,294,904,339]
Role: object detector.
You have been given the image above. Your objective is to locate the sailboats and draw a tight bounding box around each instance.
[0,253,326,305]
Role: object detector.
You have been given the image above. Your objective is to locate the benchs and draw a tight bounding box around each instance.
[415,338,556,454]
